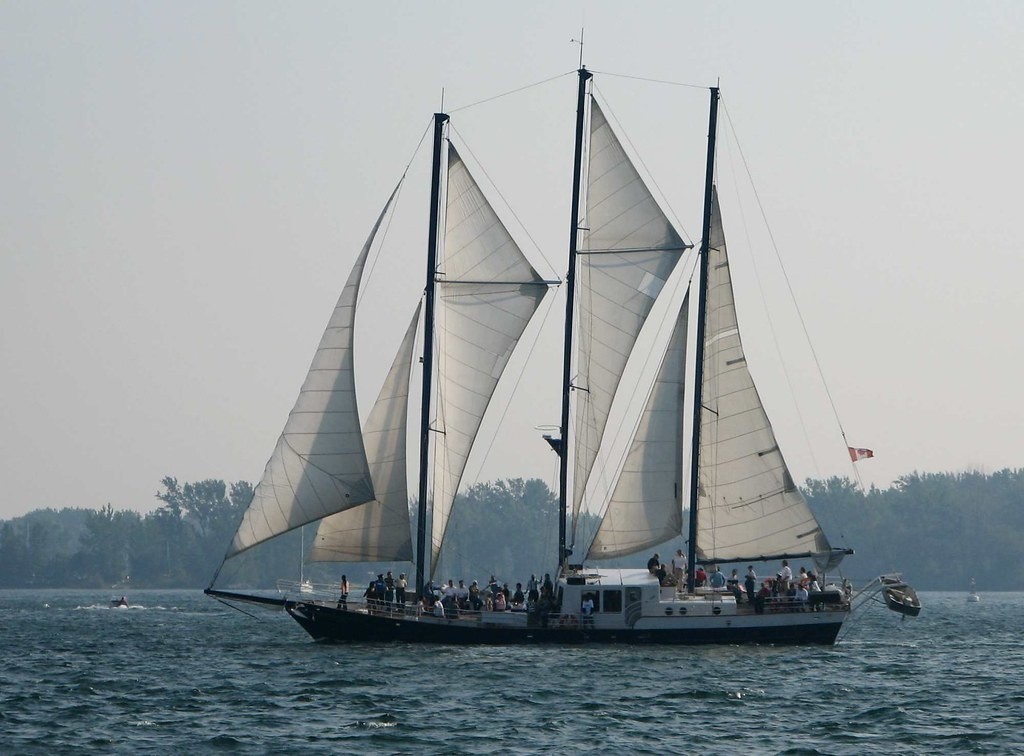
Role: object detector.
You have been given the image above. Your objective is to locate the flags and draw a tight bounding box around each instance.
[848,447,874,462]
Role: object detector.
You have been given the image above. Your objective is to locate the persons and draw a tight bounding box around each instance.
[416,574,524,618]
[745,560,821,614]
[727,569,743,604]
[581,595,595,629]
[709,566,726,588]
[648,549,688,593]
[363,572,407,614]
[695,568,708,587]
[337,575,349,610]
[523,573,557,629]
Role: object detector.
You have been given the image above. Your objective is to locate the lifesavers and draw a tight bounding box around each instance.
[560,614,579,629]
[486,598,494,611]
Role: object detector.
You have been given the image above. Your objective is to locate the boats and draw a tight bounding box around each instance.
[111,598,128,607]
[881,577,922,616]
[966,595,979,603]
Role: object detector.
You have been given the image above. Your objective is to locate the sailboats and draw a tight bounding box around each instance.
[204,27,855,644]
